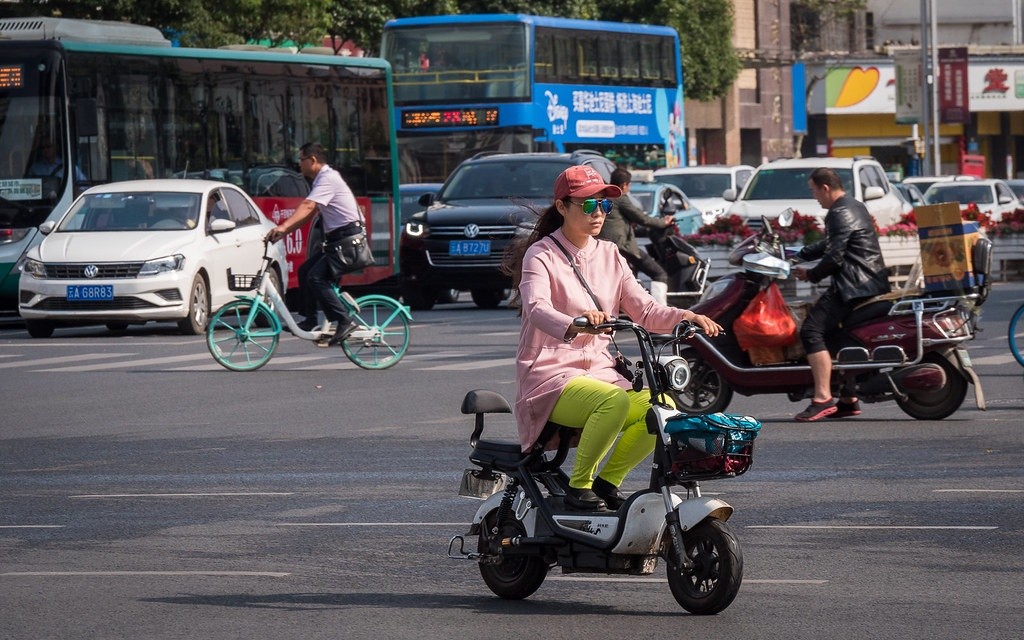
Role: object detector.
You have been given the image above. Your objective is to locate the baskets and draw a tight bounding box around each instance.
[226,267,263,291]
[665,414,762,482]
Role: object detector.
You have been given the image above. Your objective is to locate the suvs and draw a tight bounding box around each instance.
[400,148,617,311]
[722,154,913,229]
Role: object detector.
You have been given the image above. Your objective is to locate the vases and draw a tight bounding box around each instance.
[695,231,918,297]
[990,236,1024,260]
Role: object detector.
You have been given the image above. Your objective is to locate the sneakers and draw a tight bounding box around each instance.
[824,399,861,418]
[795,397,838,422]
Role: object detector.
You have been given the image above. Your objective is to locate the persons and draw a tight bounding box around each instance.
[27,136,88,194]
[793,167,891,422]
[265,142,366,347]
[185,194,219,231]
[514,165,724,512]
[594,167,677,307]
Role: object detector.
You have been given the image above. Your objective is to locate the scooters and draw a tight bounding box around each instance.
[446,315,759,614]
[663,207,995,421]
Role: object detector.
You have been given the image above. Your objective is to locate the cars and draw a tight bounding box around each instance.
[18,177,289,336]
[902,176,976,194]
[653,165,755,224]
[627,179,704,237]
[395,184,443,233]
[913,180,1024,223]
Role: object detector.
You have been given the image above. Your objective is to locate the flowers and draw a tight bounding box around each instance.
[961,203,1024,235]
[683,211,918,248]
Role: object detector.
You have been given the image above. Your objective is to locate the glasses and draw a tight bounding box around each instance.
[298,157,310,162]
[566,198,614,216]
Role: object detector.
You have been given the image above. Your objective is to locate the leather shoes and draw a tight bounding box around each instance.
[563,489,608,511]
[282,321,318,332]
[328,318,359,347]
[591,484,628,510]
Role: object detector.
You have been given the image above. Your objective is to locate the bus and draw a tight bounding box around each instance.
[379,11,687,229]
[0,18,401,311]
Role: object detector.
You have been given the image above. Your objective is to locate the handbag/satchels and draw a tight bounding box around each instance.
[320,226,376,280]
[614,350,635,382]
[733,280,797,351]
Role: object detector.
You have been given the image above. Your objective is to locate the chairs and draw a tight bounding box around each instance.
[115,198,149,224]
[398,62,663,98]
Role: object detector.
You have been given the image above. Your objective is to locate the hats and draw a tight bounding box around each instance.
[554,165,622,202]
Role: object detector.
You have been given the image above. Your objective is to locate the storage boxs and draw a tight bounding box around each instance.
[913,205,989,294]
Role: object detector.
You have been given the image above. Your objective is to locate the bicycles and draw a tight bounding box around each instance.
[207,238,414,371]
[1008,304,1024,367]
